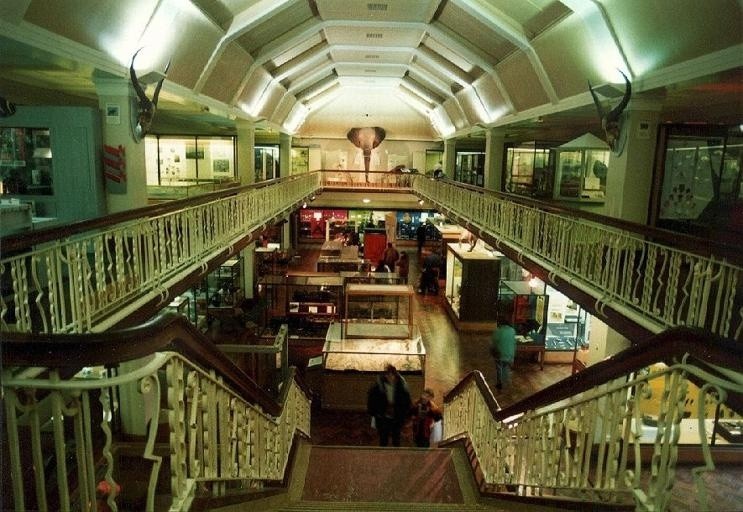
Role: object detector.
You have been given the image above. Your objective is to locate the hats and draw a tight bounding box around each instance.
[385,365,396,375]
[423,388,435,397]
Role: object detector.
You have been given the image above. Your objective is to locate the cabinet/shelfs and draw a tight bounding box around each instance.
[313,283,428,413]
[426,216,591,371]
[170,242,402,333]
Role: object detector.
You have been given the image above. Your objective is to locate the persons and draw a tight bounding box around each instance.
[416,221,426,256]
[366,219,375,228]
[374,242,409,285]
[434,159,442,172]
[490,316,516,389]
[337,229,364,257]
[404,388,443,448]
[366,364,412,447]
[419,248,443,295]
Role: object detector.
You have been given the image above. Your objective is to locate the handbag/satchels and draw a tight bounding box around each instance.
[429,419,442,442]
